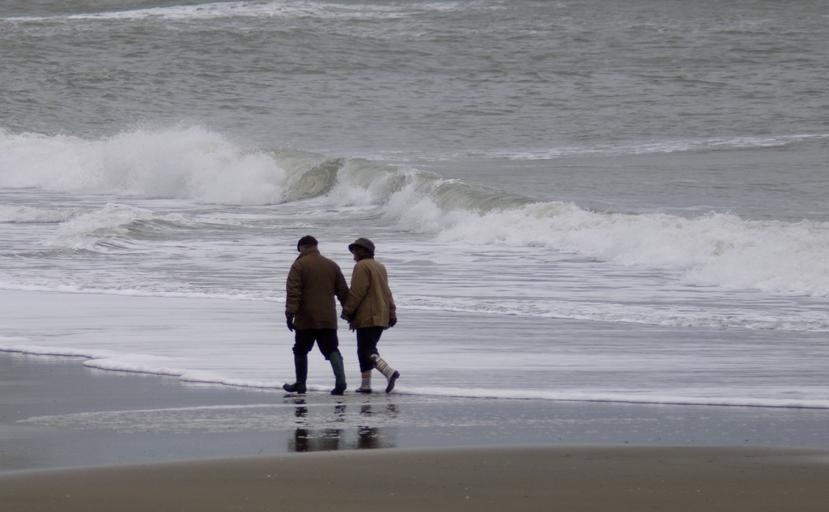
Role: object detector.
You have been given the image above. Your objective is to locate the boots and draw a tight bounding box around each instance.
[369,352,400,393]
[355,369,372,394]
[330,351,347,396]
[282,352,308,394]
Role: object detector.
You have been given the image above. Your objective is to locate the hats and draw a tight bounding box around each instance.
[348,237,375,257]
[297,235,317,252]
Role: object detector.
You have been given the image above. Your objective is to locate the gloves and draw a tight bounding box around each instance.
[285,312,295,332]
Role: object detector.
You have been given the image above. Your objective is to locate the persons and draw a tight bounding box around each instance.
[283,235,350,394]
[341,238,400,393]
